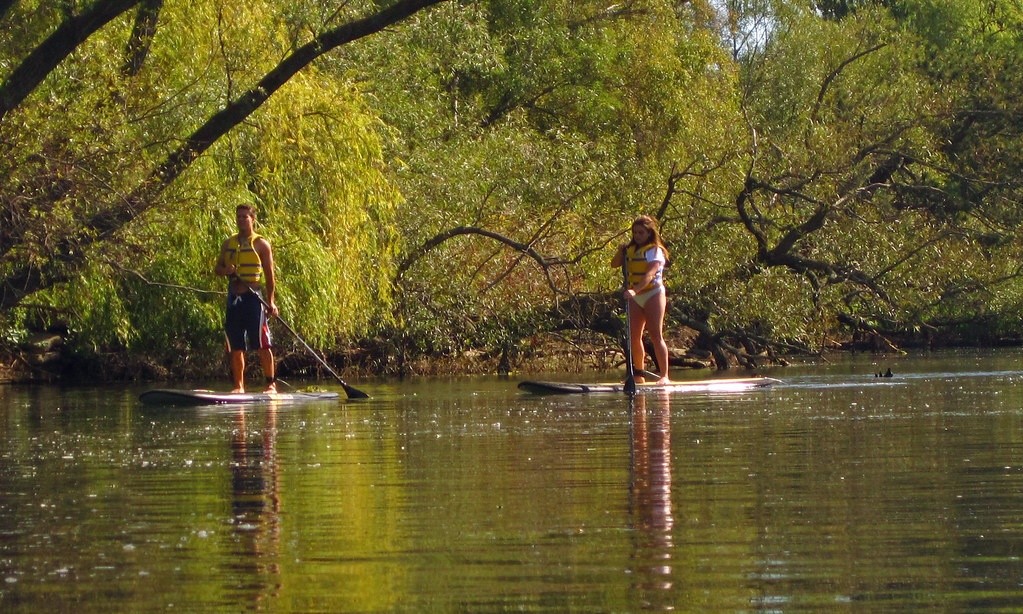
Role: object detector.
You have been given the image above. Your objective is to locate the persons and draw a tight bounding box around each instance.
[215,203,279,393]
[611,215,670,384]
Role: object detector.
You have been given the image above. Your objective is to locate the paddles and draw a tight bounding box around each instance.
[621,247,639,397]
[232,270,368,399]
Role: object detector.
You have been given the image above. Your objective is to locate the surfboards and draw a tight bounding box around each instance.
[140,388,343,406]
[516,379,775,396]
